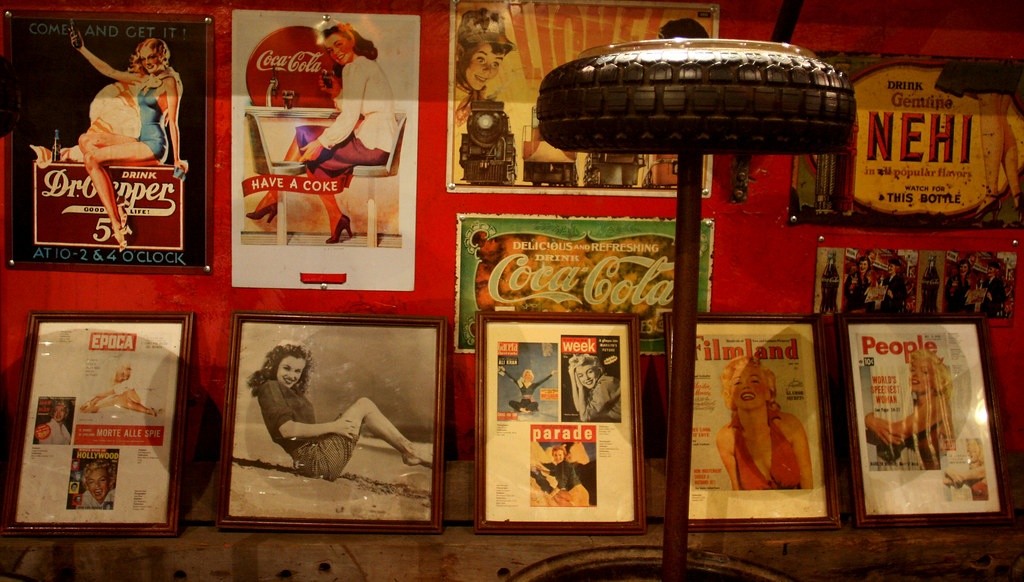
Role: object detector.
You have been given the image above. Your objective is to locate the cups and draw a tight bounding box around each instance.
[283,91,295,109]
[322,70,334,89]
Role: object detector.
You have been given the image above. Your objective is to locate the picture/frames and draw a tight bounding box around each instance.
[836,313,1018,530]
[663,309,842,532]
[0,308,196,537]
[216,309,449,535]
[474,309,647,536]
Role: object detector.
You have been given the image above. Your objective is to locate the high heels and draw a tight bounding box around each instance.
[1014,192,1024,221]
[326,215,353,244]
[968,194,1002,223]
[245,203,277,224]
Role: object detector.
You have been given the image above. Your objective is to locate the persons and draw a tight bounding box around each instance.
[38,399,71,446]
[538,446,590,506]
[500,366,555,415]
[864,350,957,472]
[246,344,433,481]
[942,440,988,501]
[715,355,812,491]
[568,354,622,422]
[78,459,115,511]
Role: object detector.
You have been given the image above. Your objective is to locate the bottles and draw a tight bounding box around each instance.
[919,254,940,313]
[820,250,839,315]
[952,277,958,295]
[70,18,82,49]
[852,273,858,287]
[52,130,61,162]
